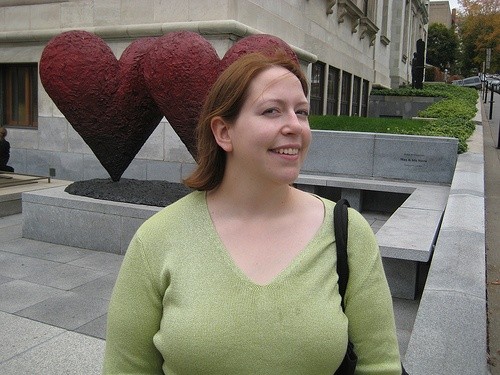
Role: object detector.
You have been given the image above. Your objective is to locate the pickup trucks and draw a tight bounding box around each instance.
[452,76,482,91]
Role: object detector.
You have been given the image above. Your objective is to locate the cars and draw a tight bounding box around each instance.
[481,74,500,93]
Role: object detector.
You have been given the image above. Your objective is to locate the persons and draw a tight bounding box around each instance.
[412,52,424,90]
[103,52,402,375]
[0,128,14,172]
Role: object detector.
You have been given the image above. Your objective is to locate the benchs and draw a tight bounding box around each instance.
[293,171,451,300]
